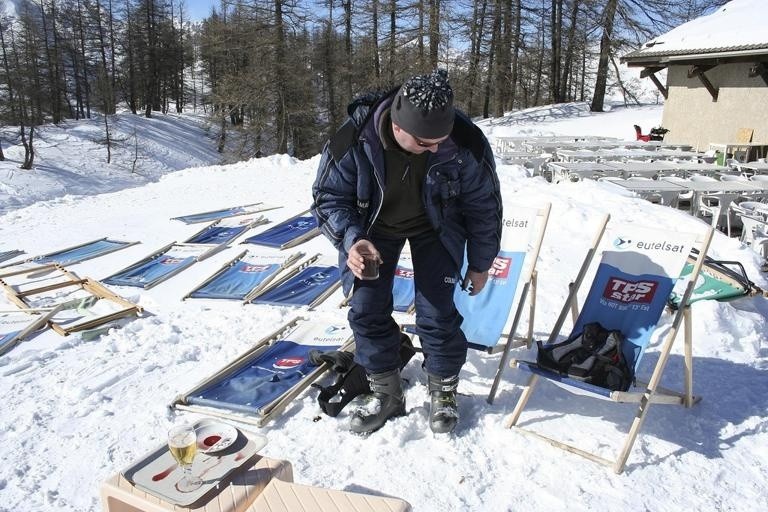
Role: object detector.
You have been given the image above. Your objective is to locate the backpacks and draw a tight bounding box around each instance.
[537,322,635,390]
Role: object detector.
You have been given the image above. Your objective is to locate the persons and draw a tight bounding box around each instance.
[308,67,506,437]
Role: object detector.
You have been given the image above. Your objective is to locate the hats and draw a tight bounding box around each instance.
[390,74,456,139]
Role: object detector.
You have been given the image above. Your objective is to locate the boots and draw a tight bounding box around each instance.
[349,368,407,433]
[427,373,459,433]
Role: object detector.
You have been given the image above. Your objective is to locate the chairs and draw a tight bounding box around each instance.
[489,125,768,261]
[503,212,715,476]
[0,198,555,432]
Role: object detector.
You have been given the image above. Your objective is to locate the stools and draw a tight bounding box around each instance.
[100,453,294,512]
[241,475,414,511]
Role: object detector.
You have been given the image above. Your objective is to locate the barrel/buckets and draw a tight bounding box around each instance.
[735,150,746,163]
[716,151,724,166]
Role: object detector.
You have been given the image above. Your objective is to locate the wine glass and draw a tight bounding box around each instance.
[163,424,203,493]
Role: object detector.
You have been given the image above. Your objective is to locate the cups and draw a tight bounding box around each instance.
[359,250,382,280]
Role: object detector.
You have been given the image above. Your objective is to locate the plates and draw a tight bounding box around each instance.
[192,423,238,455]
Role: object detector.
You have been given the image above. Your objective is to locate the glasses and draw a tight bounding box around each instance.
[415,136,447,148]
